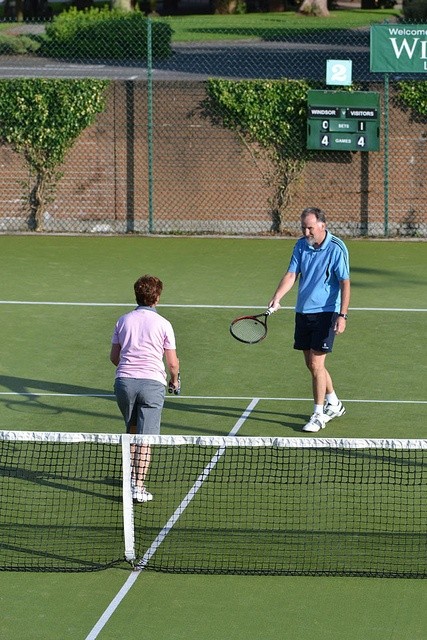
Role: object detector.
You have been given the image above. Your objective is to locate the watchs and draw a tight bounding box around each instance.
[339,314,349,320]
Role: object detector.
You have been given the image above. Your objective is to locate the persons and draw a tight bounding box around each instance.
[267,206,352,432]
[109,274,182,504]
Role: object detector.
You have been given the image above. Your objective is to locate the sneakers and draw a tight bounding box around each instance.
[131,479,153,502]
[303,412,326,432]
[324,401,346,422]
